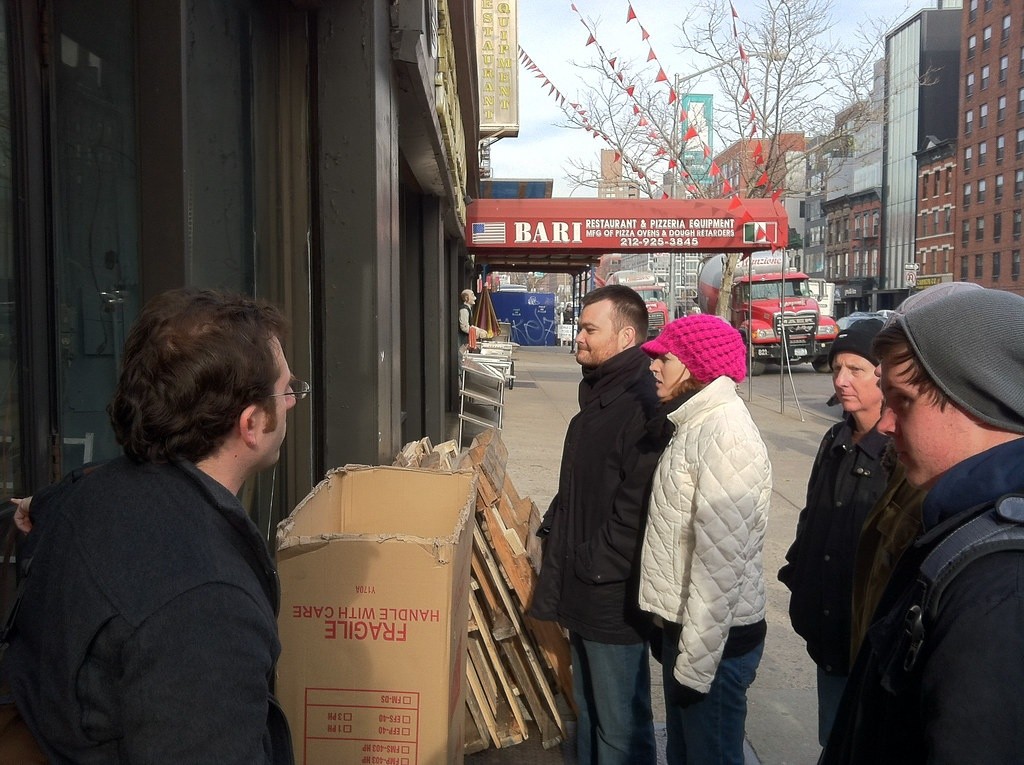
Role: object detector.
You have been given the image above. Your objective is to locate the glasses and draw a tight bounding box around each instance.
[238,376,310,414]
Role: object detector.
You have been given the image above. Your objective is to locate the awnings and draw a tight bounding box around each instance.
[467,197,788,414]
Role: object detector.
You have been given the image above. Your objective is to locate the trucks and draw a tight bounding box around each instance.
[695,251,840,378]
[602,270,668,341]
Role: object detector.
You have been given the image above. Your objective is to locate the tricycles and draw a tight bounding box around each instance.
[469,319,515,390]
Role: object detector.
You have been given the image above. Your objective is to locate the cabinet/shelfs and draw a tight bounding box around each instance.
[458,353,510,452]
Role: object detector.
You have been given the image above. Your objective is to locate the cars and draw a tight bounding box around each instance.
[836,308,897,329]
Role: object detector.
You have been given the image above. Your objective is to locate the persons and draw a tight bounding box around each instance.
[528,285,664,765]
[634,313,774,765]
[0,289,311,764]
[778,283,1024,764]
[458,289,487,346]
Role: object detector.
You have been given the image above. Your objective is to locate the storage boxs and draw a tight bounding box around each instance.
[273,463,479,765]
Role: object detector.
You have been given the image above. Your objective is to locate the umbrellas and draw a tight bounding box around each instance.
[474,288,500,342]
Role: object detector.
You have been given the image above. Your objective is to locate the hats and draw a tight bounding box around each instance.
[881,282,984,330]
[828,318,884,367]
[641,314,747,383]
[896,289,1024,432]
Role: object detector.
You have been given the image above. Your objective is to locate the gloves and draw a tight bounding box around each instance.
[671,680,706,708]
[648,624,663,664]
